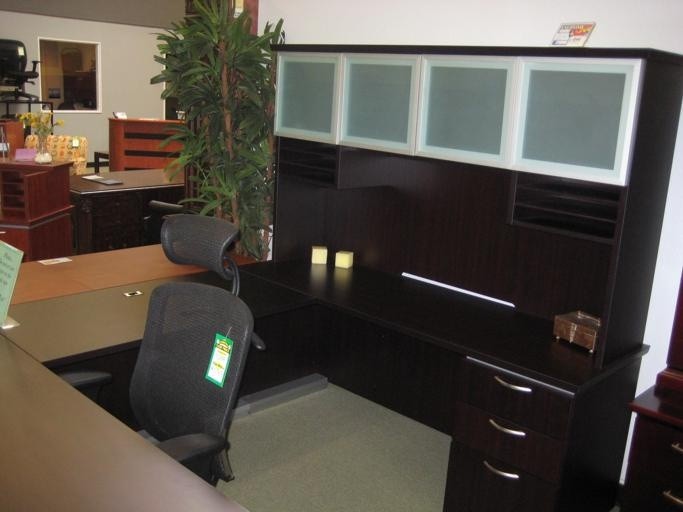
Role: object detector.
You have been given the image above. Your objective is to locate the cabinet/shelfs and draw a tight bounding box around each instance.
[621,388,683,511]
[442,322,650,511]
[0,157,76,262]
[271,43,683,370]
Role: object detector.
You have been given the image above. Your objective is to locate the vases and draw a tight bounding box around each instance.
[35,135,51,164]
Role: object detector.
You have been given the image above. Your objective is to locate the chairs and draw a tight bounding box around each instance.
[0,39,43,101]
[129,213,265,489]
[23,136,88,176]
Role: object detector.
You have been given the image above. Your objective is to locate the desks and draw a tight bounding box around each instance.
[238,258,551,436]
[67,166,186,255]
[3,268,315,432]
[0,335,252,512]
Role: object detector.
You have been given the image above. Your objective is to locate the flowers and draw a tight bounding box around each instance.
[16,111,66,152]
